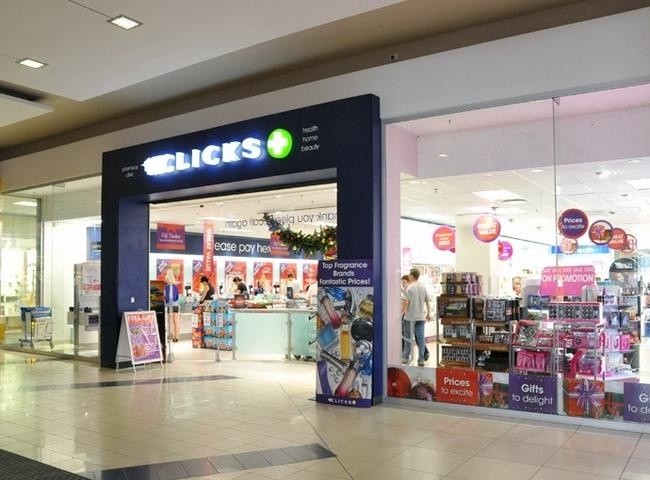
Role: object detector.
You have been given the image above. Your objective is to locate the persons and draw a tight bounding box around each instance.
[611,270,627,283]
[233,277,247,294]
[402,268,432,366]
[288,272,299,294]
[165,273,180,342]
[199,276,215,304]
[512,276,525,299]
[402,274,430,361]
[255,281,266,296]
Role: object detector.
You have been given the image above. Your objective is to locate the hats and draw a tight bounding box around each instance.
[233,277,242,282]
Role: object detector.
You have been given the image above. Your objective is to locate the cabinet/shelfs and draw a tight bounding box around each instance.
[191,296,319,364]
[435,282,649,383]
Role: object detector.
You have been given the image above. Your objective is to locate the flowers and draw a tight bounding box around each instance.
[261,211,336,257]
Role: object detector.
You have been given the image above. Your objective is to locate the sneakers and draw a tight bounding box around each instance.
[173,336,178,342]
[401,352,429,367]
